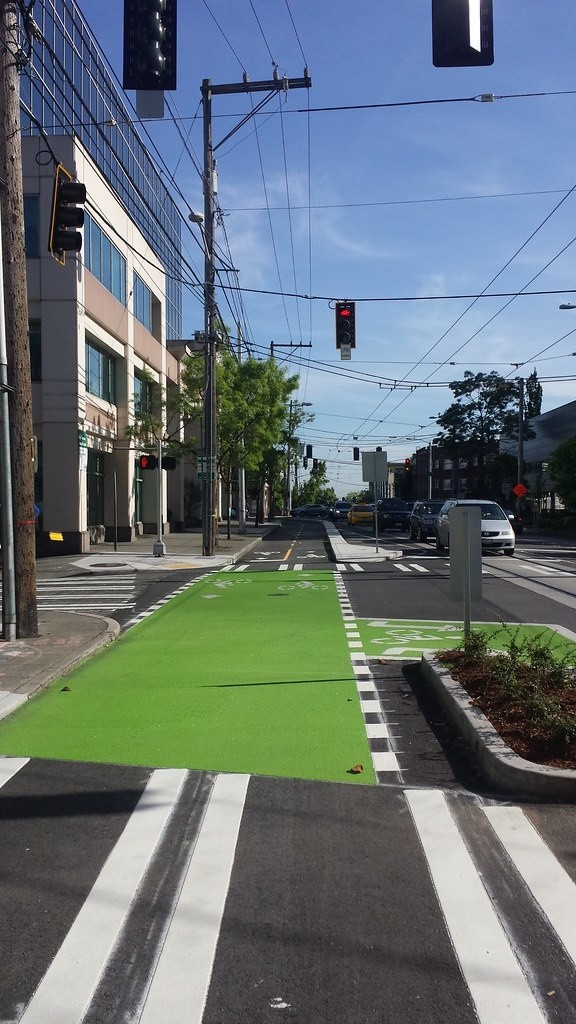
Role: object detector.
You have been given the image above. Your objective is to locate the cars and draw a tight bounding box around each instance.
[292,505,310,517]
[347,503,374,527]
[330,501,353,522]
[504,510,524,534]
[298,504,329,518]
[435,499,516,556]
[409,501,446,542]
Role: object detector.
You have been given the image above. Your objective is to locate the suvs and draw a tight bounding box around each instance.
[373,499,412,533]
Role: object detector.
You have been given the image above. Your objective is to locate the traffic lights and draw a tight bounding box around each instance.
[164,456,176,470]
[139,455,156,470]
[336,302,356,349]
[48,165,86,266]
[405,459,410,471]
[304,457,308,467]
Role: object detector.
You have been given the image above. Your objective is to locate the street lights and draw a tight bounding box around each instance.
[285,401,314,517]
[406,438,432,499]
[189,212,215,555]
